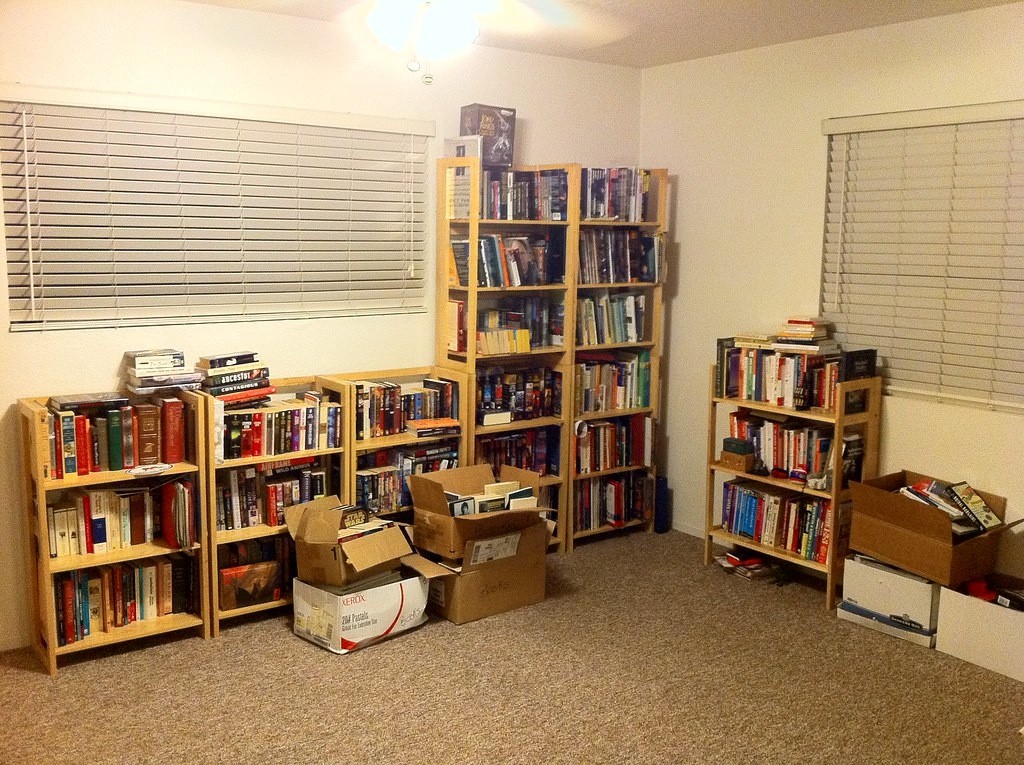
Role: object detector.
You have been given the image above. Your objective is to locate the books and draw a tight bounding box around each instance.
[27,135,668,646]
[713,316,1024,648]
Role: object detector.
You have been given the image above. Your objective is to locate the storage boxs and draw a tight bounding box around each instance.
[606,480,623,528]
[632,473,654,524]
[283,463,559,654]
[837,469,1024,683]
[721,437,755,474]
[457,103,516,168]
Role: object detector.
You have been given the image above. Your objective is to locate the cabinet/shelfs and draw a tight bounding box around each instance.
[704,364,882,612]
[435,158,668,557]
[17,365,470,677]
